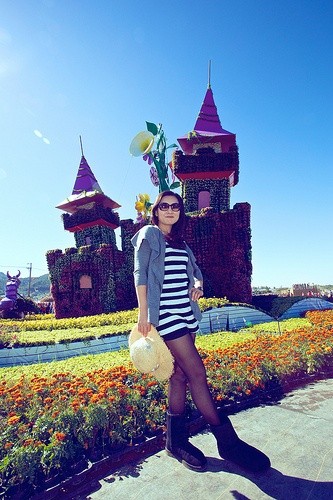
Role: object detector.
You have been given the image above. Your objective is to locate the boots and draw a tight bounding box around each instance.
[210,414,271,474]
[165,409,207,472]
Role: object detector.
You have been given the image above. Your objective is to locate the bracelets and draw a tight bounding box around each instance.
[197,287,203,291]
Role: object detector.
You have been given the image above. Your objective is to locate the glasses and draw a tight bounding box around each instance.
[158,202,180,212]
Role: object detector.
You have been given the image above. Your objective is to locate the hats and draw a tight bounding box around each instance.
[129,323,175,381]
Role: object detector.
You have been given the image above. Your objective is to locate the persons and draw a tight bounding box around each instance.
[131,190,271,473]
